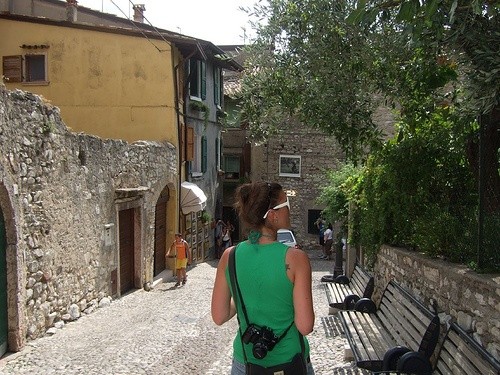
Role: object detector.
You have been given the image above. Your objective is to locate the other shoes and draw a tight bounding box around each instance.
[182,278,187,285]
[175,282,181,286]
[320,254,330,260]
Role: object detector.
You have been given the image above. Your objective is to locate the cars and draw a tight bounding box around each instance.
[276,228,299,250]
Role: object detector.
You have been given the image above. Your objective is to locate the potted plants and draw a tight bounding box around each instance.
[189,101,209,131]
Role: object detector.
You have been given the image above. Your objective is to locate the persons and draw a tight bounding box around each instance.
[317,217,329,259]
[211,181,315,375]
[324,223,333,260]
[214,216,234,259]
[170,233,191,286]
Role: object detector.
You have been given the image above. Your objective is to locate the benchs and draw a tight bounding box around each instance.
[340,278,441,375]
[396,322,500,375]
[323,261,374,309]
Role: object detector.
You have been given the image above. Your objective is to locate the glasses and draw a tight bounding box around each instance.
[263,197,290,218]
[267,182,282,209]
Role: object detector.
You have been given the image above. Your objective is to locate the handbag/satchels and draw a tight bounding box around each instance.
[245,354,307,375]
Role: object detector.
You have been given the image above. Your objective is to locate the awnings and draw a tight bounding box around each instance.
[180,181,207,215]
